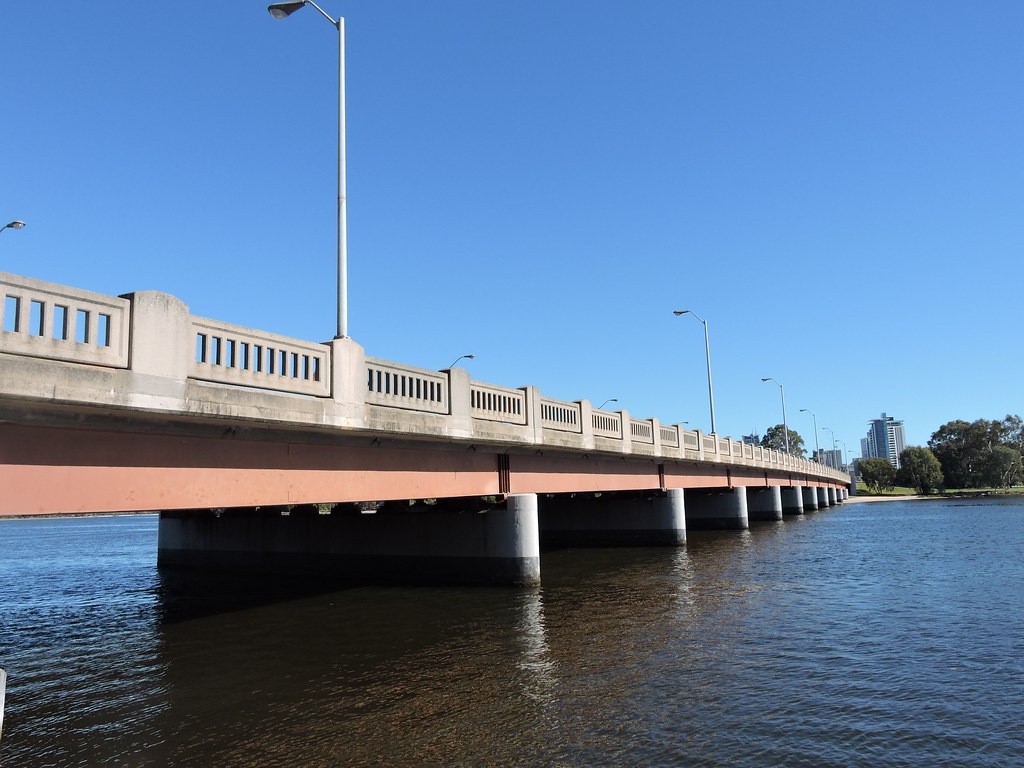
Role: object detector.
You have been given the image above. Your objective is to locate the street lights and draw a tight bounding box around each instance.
[267,2,346,338]
[800,409,820,463]
[762,377,790,454]
[835,440,847,473]
[849,449,861,464]
[674,310,715,434]
[822,427,837,469]
